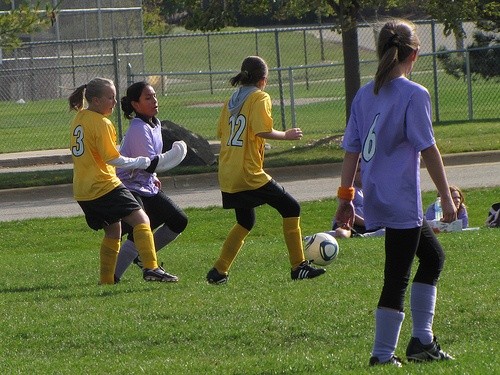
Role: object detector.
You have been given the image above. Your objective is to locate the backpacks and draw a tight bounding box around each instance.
[486,204,500,227]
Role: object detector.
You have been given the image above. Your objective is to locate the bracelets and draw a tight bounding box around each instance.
[338,187,355,200]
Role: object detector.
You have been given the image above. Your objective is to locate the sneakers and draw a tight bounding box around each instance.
[405,334,456,364]
[133,255,144,268]
[290,259,326,280]
[207,268,229,287]
[369,354,406,368]
[142,262,179,282]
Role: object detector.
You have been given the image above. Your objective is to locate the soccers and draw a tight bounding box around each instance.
[304,233,339,266]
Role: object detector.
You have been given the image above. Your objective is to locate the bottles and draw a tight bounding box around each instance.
[434,197,444,221]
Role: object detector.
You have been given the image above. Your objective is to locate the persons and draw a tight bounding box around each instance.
[111,83,190,283]
[423,185,468,234]
[325,155,393,240]
[66,78,179,287]
[328,17,460,366]
[202,56,325,285]
